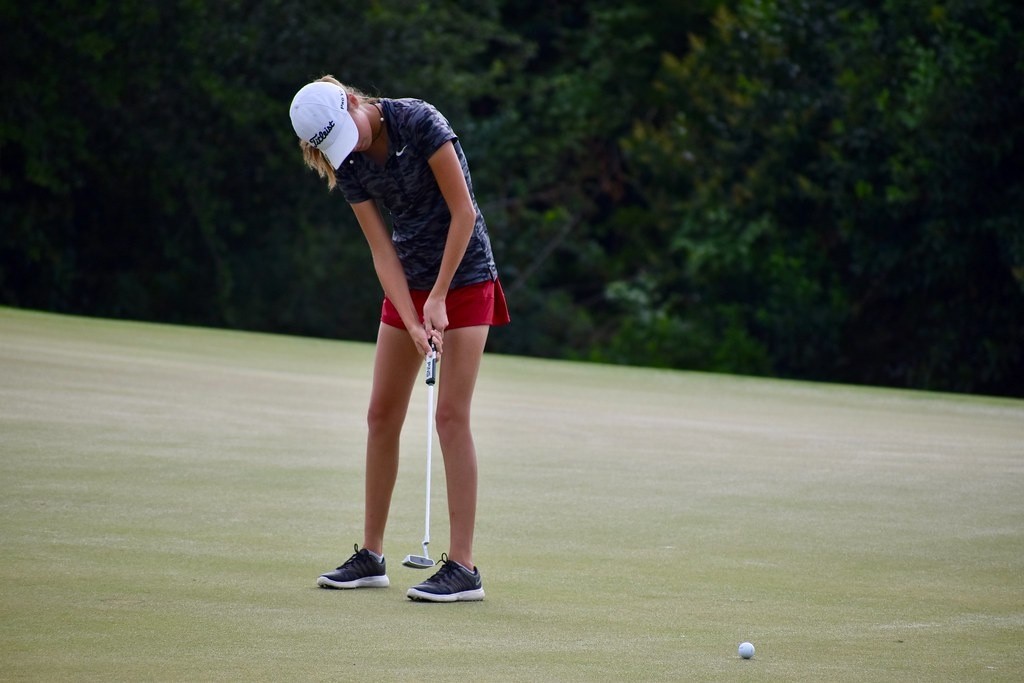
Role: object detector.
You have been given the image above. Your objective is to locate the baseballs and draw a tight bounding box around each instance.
[738,641,756,660]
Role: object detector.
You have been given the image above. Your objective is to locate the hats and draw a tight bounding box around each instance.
[289,82,360,170]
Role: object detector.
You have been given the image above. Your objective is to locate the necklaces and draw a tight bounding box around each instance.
[370,102,384,143]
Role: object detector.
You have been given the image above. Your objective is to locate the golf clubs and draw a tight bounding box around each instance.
[400,337,438,571]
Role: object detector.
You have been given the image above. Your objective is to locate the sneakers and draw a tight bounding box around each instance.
[407,552,486,603]
[316,544,391,588]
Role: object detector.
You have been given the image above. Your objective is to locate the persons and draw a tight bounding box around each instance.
[289,74,509,602]
